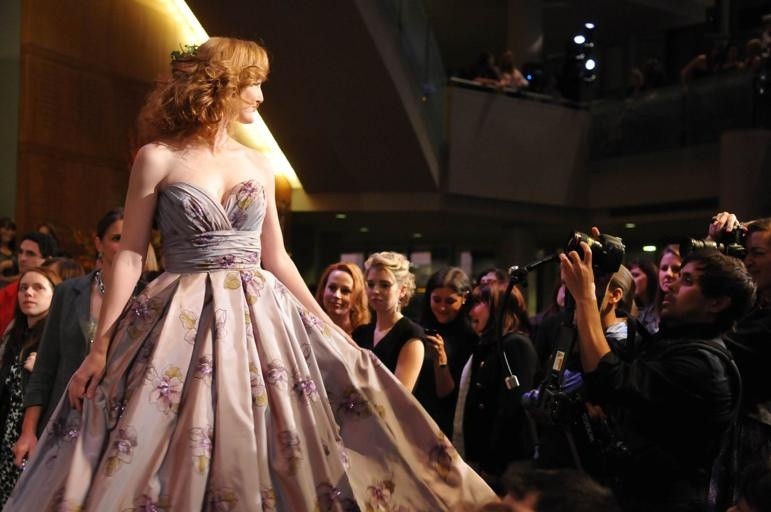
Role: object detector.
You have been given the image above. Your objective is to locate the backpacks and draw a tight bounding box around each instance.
[657,337,771,512]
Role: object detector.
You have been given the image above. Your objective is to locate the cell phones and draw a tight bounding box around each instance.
[424,328,437,342]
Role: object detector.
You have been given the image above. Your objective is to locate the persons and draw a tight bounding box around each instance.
[1,205,163,509]
[316,263,370,336]
[352,251,428,393]
[415,211,770,512]
[2,35,508,509]
[624,15,769,102]
[469,48,528,91]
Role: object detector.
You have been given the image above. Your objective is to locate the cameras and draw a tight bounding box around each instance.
[679,225,747,260]
[562,232,622,327]
[519,374,580,459]
